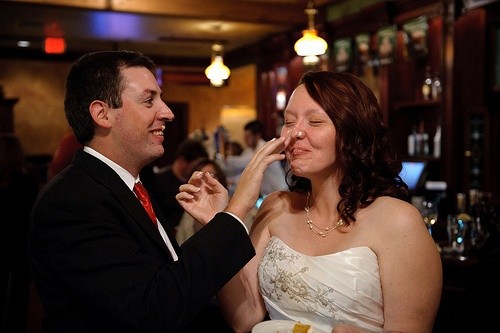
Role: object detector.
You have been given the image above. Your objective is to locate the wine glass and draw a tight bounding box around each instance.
[421,200,438,236]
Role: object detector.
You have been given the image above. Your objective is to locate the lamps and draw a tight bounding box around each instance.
[293,0,328,66]
[205,43,230,88]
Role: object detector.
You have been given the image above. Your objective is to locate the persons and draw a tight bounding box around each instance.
[26,50,291,333]
[175,71,444,333]
[46,121,292,247]
[0,135,42,333]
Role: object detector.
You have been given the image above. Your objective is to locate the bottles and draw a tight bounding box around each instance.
[456,188,500,246]
[421,66,441,102]
[407,115,441,158]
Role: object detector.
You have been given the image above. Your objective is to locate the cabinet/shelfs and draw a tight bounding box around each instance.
[257,4,448,196]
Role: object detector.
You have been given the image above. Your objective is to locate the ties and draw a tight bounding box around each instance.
[134,183,157,227]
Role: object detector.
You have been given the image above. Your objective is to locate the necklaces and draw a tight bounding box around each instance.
[304,191,344,237]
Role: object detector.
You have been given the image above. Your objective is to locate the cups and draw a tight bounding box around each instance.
[252,321,310,333]
[448,218,463,249]
